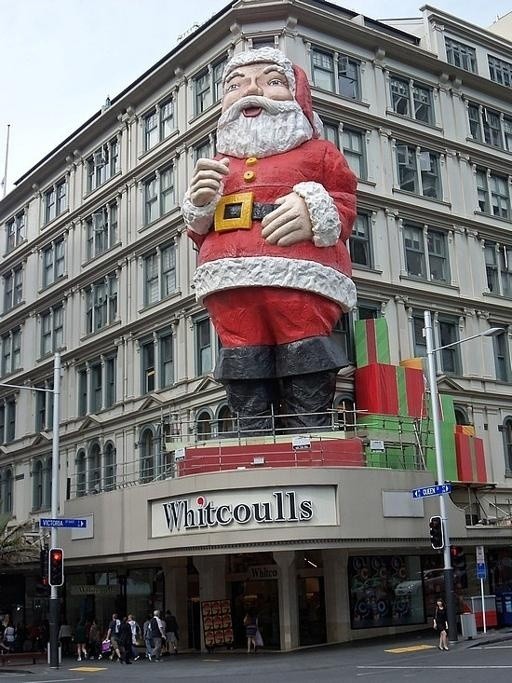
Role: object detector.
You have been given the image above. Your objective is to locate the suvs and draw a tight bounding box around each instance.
[350,575,396,599]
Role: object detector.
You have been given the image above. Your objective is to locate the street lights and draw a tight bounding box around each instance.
[421,307,508,641]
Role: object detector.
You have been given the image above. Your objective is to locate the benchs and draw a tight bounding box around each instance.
[0,652,45,667]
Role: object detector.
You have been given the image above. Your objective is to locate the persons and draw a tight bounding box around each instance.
[433,598,449,651]
[57,609,180,664]
[181,46,359,431]
[0,610,50,654]
[243,606,259,653]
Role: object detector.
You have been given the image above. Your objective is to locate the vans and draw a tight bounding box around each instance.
[395,566,460,598]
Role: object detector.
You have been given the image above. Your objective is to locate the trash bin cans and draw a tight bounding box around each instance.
[497,592,512,628]
[465,594,498,628]
[47,640,62,667]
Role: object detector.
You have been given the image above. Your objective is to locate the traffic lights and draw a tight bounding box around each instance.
[49,546,65,586]
[429,515,444,548]
[39,549,48,584]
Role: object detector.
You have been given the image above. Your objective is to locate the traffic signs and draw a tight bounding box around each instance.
[40,518,86,528]
[412,482,452,500]
